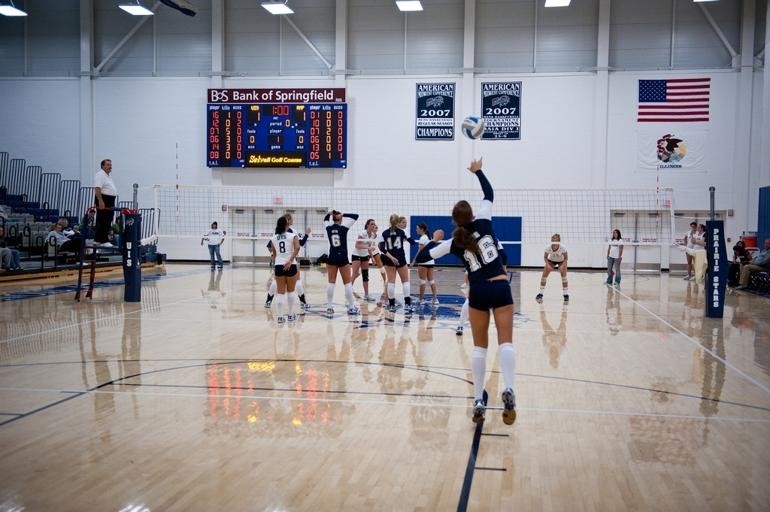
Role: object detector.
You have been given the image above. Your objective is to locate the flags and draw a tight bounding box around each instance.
[638,78,711,123]
[638,126,708,174]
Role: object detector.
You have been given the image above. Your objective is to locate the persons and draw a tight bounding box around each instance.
[354,226,388,300]
[42,205,97,265]
[381,217,416,299]
[691,223,708,285]
[380,214,415,312]
[416,156,517,420]
[324,209,358,319]
[684,222,699,280]
[93,159,114,248]
[604,229,624,286]
[408,223,440,305]
[201,222,225,271]
[264,212,313,308]
[378,216,408,300]
[455,240,508,336]
[112,216,122,247]
[536,234,570,302]
[0,214,24,275]
[727,238,770,290]
[351,220,375,302]
[271,217,300,323]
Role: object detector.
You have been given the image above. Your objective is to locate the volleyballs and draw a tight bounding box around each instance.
[462,114,487,140]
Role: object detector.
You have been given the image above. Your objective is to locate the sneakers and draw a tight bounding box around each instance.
[432,298,439,305]
[389,307,396,313]
[735,287,739,290]
[300,303,310,309]
[363,296,375,301]
[472,399,486,422]
[689,276,696,281]
[354,294,360,299]
[536,293,543,299]
[614,281,620,285]
[326,309,334,319]
[93,240,102,246]
[502,388,516,425]
[377,301,387,308]
[288,313,296,320]
[683,276,692,280]
[100,242,114,247]
[211,265,215,269]
[738,286,747,289]
[5,267,16,270]
[277,317,285,323]
[405,306,417,314]
[16,266,23,271]
[75,262,88,265]
[604,282,612,284]
[456,326,464,336]
[218,266,222,270]
[564,295,569,301]
[265,302,271,307]
[348,308,358,315]
[416,299,426,305]
